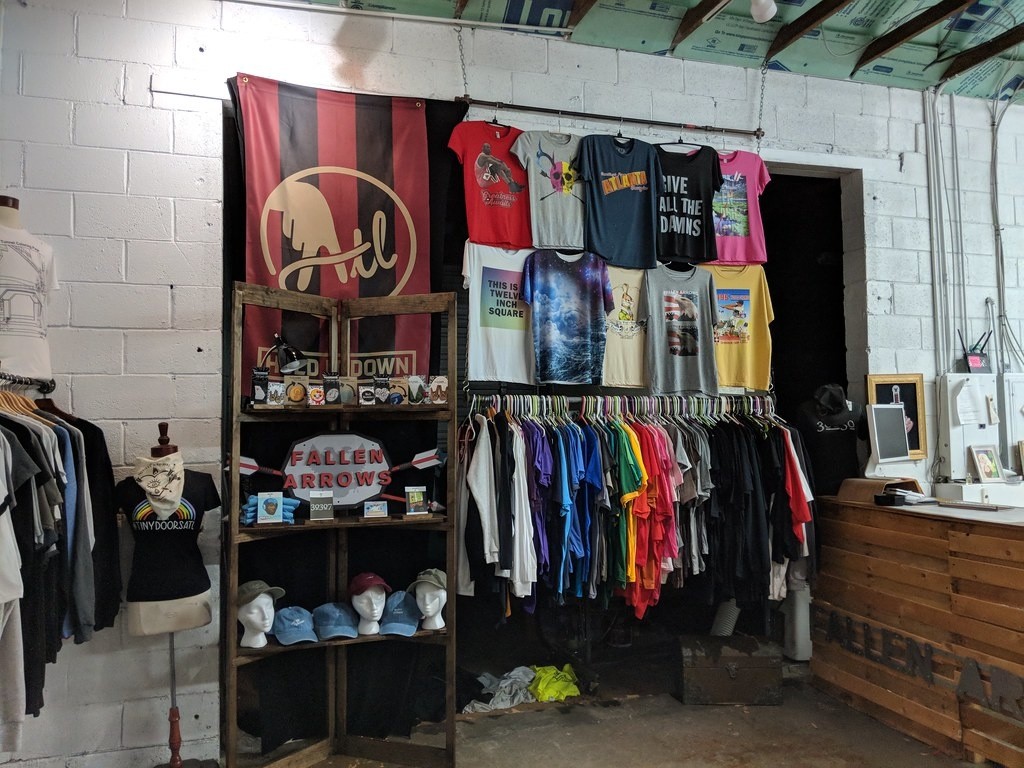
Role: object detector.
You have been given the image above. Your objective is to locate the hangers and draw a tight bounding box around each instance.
[550,109,570,137]
[0,372,77,428]
[457,393,788,441]
[614,116,633,141]
[485,101,507,127]
[716,128,736,151]
[666,259,694,270]
[660,124,703,146]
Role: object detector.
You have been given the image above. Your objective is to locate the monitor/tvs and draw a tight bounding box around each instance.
[866,403,910,480]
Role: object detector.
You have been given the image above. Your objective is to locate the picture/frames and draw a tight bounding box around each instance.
[868,373,928,460]
[970,444,1007,483]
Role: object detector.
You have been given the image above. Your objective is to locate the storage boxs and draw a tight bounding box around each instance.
[680,634,784,706]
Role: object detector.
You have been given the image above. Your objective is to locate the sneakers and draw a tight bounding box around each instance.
[509,181,527,192]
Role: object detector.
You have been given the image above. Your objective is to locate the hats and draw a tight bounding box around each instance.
[348,572,392,596]
[273,606,318,645]
[313,602,359,639]
[406,568,447,592]
[238,580,286,608]
[379,591,422,637]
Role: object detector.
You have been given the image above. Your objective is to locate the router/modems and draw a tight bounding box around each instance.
[957,328,993,374]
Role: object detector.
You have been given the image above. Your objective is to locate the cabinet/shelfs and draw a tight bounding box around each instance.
[226,281,458,768]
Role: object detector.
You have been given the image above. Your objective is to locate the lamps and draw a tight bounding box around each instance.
[252,333,307,373]
[751,0,777,23]
